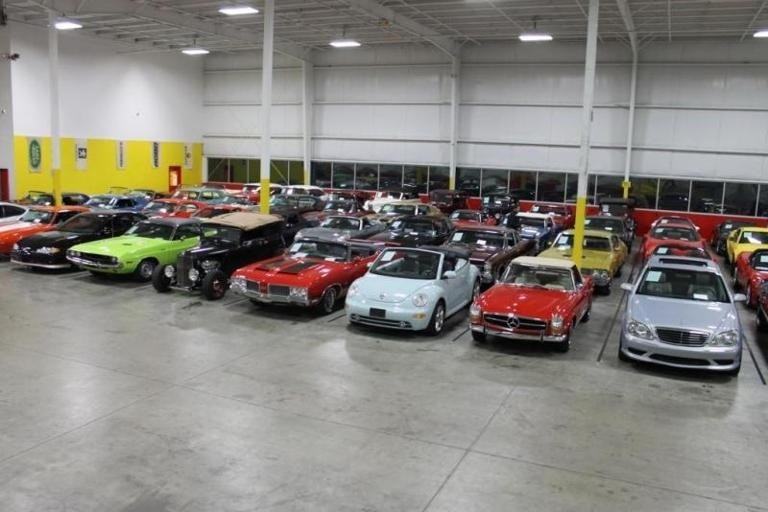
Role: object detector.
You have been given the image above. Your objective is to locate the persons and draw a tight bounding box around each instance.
[484,213,498,226]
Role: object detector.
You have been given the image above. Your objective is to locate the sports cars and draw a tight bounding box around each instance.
[344,246,484,336]
[228,237,409,315]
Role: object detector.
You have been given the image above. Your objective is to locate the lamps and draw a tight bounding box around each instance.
[180,39,210,55]
[516,16,554,42]
[328,30,362,48]
[217,0,260,16]
[50,13,82,32]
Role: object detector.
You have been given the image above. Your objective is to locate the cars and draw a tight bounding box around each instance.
[152,212,286,301]
[0,200,28,226]
[734,248,767,311]
[66,215,228,285]
[618,255,747,378]
[11,181,331,246]
[9,208,148,273]
[468,257,595,354]
[0,205,90,255]
[294,183,766,296]
[754,281,768,351]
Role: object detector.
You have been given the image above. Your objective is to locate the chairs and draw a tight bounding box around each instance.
[548,272,572,290]
[685,273,718,302]
[396,254,419,274]
[514,271,541,287]
[647,271,672,295]
